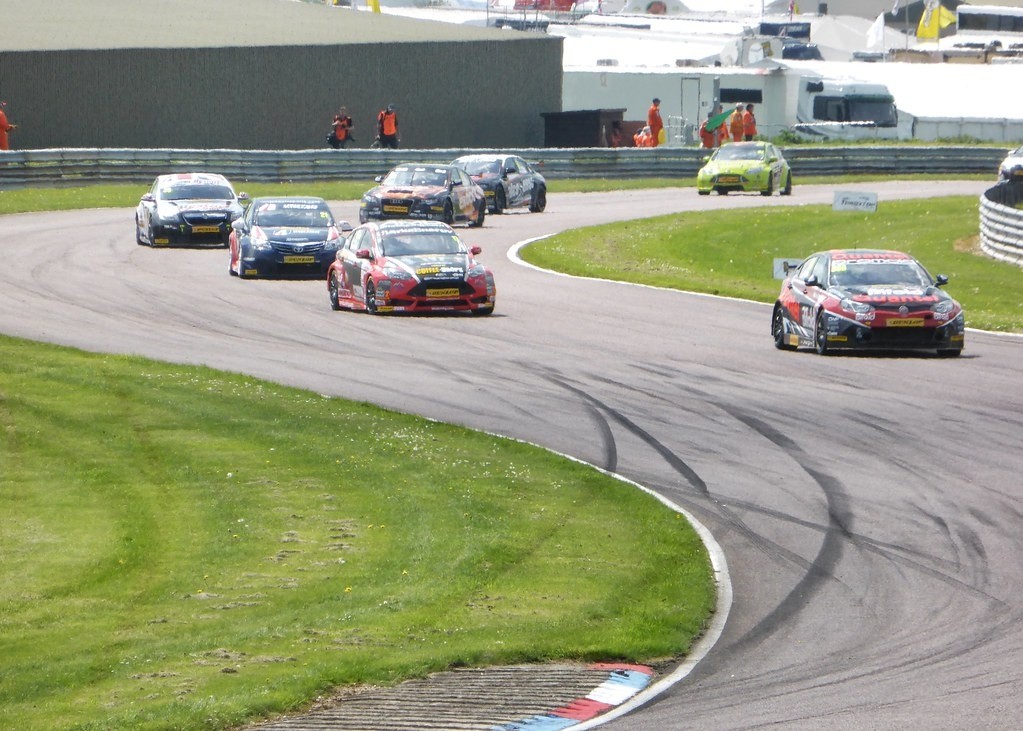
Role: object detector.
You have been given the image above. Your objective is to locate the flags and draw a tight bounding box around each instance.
[916,0,938,39]
[866,11,885,49]
[891,1,901,17]
[487,0,499,8]
[922,0,957,28]
[788,1,798,17]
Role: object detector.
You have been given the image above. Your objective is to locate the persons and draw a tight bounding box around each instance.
[729,101,744,142]
[743,103,757,142]
[719,105,728,147]
[334,106,356,148]
[331,113,348,148]
[0,101,15,150]
[649,98,665,146]
[610,120,623,146]
[699,112,714,148]
[377,103,400,148]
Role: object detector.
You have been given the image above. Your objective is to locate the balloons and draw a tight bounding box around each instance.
[633,126,653,147]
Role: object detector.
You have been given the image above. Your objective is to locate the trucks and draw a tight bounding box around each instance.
[956,5,1023,36]
[794,78,897,140]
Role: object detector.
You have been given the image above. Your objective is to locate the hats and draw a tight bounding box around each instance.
[736,103,744,108]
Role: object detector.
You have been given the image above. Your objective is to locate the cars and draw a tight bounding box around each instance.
[999,146,1023,180]
[696,141,792,197]
[359,164,486,228]
[326,219,496,315]
[136,172,248,248]
[228,195,353,280]
[450,153,547,214]
[770,248,965,357]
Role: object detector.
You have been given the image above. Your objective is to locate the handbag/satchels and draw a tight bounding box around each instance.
[326,130,337,145]
[369,139,383,149]
